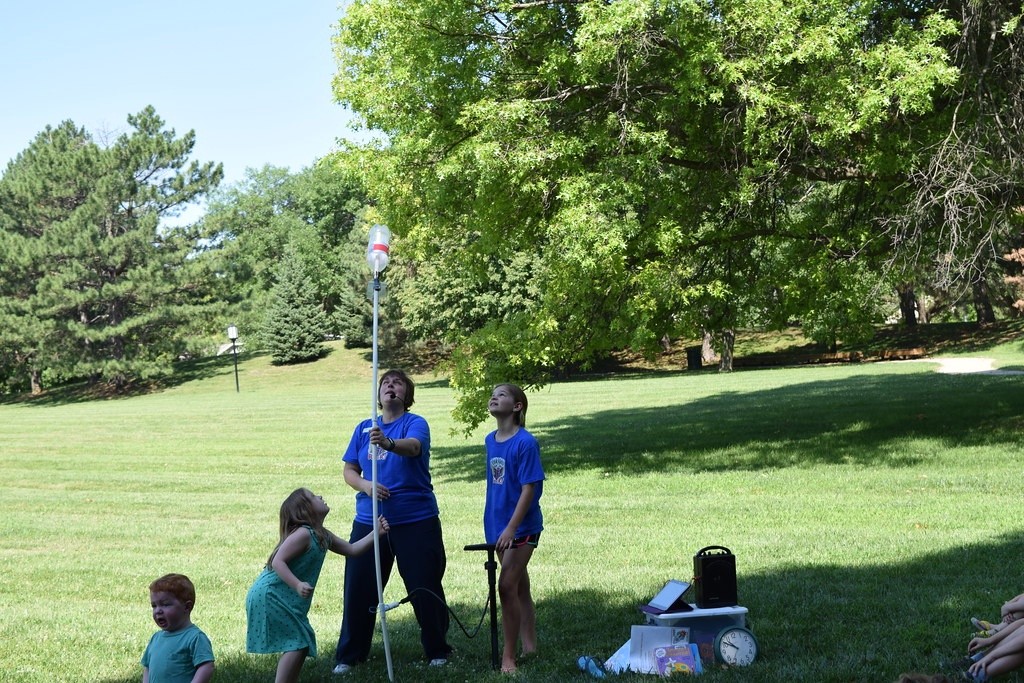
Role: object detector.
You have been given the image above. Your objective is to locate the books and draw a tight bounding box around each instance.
[654,641,703,676]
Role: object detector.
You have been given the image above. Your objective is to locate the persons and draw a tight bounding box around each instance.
[898,593,1024,683]
[332,369,450,674]
[483,384,544,676]
[140,573,215,683]
[245,488,390,683]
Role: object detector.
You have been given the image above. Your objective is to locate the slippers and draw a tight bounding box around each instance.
[577,654,606,679]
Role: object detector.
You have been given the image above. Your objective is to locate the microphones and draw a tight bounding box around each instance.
[390,394,405,404]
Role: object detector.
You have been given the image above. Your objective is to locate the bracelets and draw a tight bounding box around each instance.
[385,437,395,451]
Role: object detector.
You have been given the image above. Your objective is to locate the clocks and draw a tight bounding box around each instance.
[714,625,759,669]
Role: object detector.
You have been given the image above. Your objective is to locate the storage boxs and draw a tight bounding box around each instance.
[643,603,749,665]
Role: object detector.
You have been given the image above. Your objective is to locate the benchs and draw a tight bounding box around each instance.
[756,346,926,365]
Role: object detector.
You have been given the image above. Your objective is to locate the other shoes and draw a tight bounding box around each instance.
[971,630,991,640]
[429,659,447,668]
[951,672,974,683]
[941,657,974,673]
[333,664,352,676]
[970,618,992,629]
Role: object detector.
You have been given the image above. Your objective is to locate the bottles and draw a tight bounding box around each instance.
[368,224,390,278]
